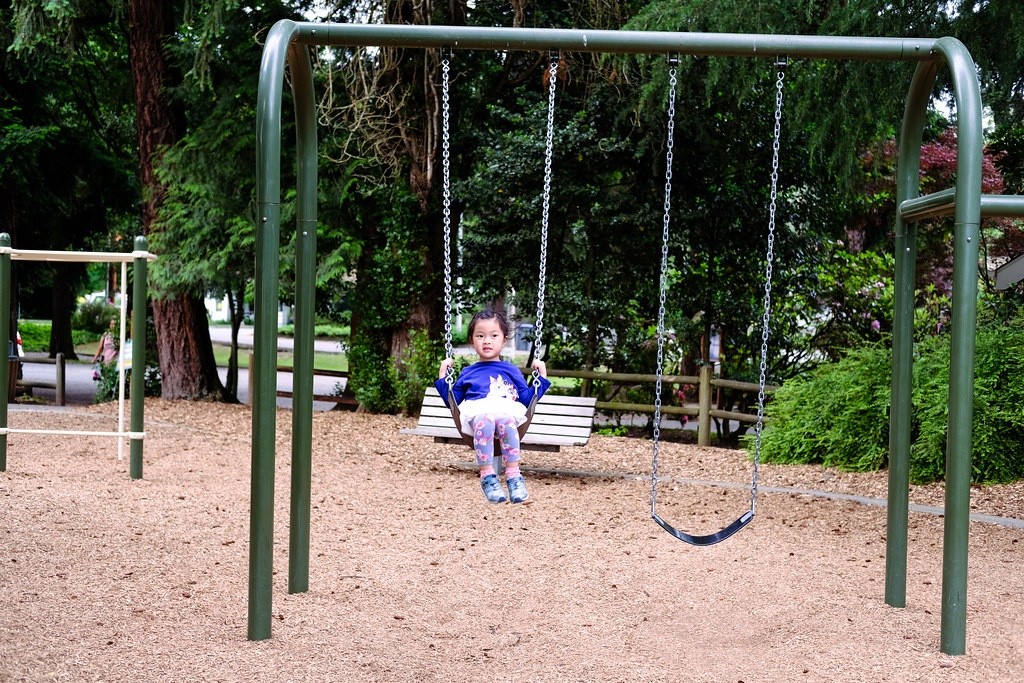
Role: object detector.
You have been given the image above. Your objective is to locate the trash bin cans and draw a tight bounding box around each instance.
[8,356,19,403]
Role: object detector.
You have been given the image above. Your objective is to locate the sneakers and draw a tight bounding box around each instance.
[506,476,529,503]
[480,473,506,503]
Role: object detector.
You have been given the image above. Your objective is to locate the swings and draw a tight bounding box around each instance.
[651,57,787,547]
[439,47,559,456]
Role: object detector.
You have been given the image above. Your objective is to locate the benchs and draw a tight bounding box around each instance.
[398,387,597,453]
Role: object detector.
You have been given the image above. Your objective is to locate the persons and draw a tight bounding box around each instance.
[92,317,118,366]
[435,309,550,504]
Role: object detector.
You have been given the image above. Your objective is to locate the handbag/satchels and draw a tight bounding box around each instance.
[92,363,100,380]
[97,354,104,362]
